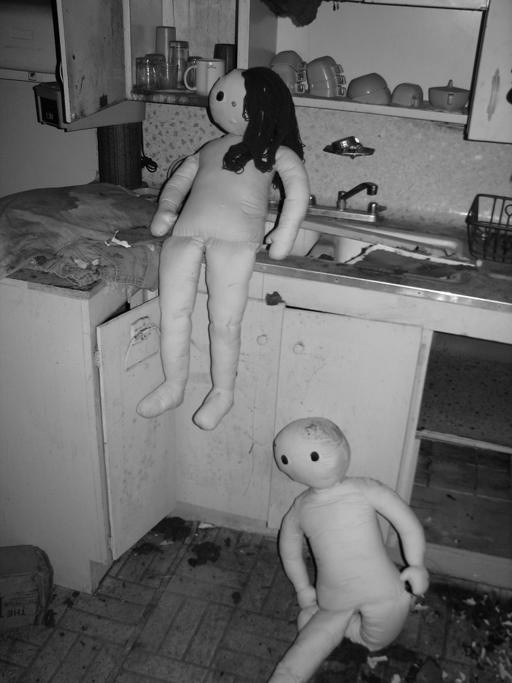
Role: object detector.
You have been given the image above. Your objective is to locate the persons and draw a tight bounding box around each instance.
[135,66,311,430]
[265,417,430,683]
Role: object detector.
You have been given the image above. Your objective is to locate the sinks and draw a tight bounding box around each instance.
[266,215,377,286]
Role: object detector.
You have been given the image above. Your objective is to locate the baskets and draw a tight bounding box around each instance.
[465,193,512,263]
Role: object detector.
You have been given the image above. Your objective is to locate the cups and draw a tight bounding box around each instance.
[271,50,346,98]
[135,26,236,97]
[392,83,423,107]
[428,86,470,111]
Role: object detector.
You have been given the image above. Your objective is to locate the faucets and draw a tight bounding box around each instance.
[336,182,377,212]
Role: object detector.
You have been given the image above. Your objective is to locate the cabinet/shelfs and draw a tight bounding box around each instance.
[57,1,512,144]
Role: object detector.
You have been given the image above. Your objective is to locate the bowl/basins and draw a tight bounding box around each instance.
[352,86,392,104]
[347,72,386,99]
